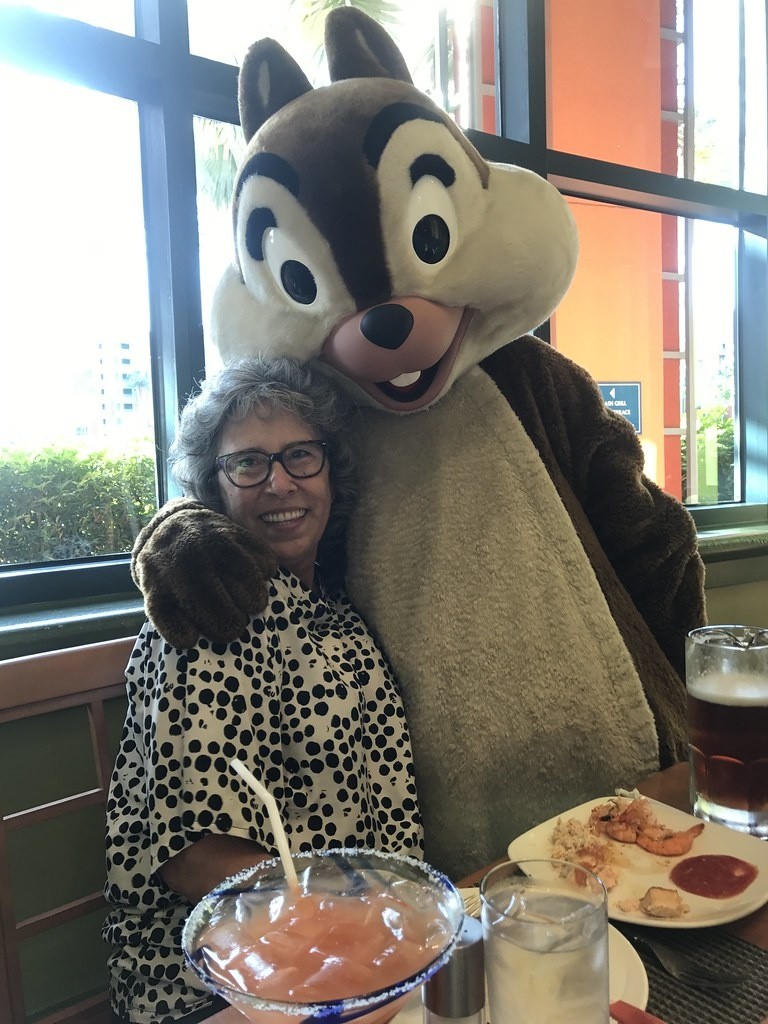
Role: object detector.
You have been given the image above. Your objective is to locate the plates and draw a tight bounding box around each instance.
[385,887,648,1024]
[507,795,768,929]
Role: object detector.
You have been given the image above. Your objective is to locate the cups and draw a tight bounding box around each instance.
[180,847,462,1024]
[480,859,610,1024]
[684,624,768,840]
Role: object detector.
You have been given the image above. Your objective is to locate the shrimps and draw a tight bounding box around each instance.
[589,797,706,857]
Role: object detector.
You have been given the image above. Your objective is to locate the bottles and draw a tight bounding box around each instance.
[421,916,485,1024]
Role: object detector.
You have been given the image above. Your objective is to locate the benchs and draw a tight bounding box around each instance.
[0,635,141,1024]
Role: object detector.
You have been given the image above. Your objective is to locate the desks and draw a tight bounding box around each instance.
[195,760,768,1024]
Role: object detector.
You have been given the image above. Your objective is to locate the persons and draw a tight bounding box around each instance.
[100,360,424,1024]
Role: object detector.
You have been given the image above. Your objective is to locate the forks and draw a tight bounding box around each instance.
[611,919,748,988]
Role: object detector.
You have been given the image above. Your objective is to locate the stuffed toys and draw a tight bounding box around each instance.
[130,4,710,881]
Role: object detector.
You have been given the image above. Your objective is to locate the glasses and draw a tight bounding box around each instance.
[213,440,329,487]
